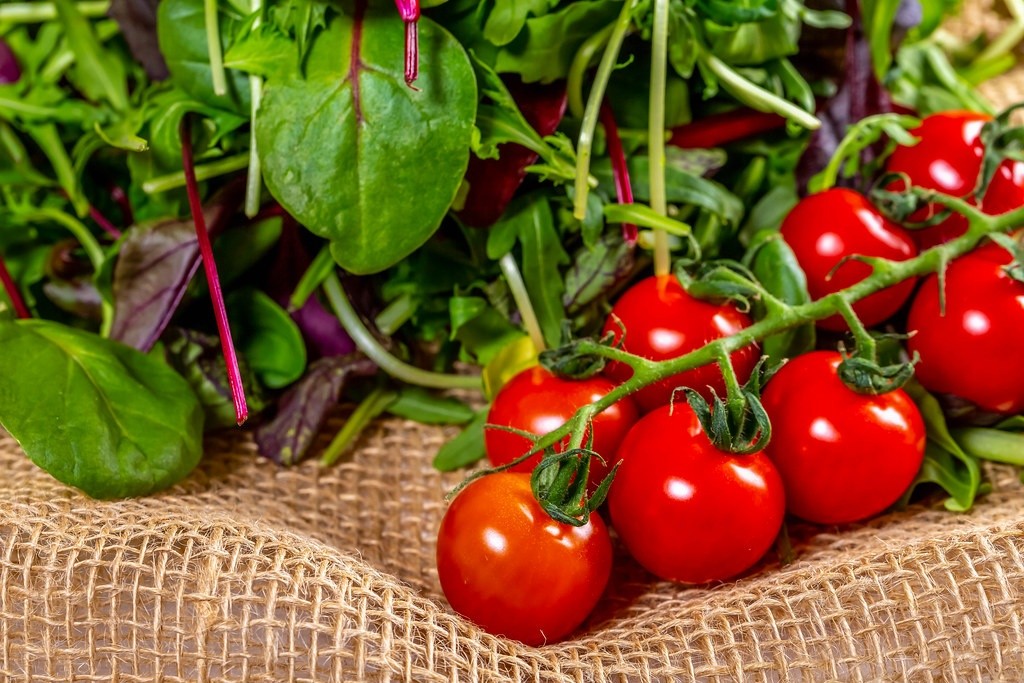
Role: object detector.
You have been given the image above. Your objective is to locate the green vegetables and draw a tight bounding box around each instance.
[0,0,1024,517]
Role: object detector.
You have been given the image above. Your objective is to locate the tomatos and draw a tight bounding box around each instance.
[436,108,1024,647]
